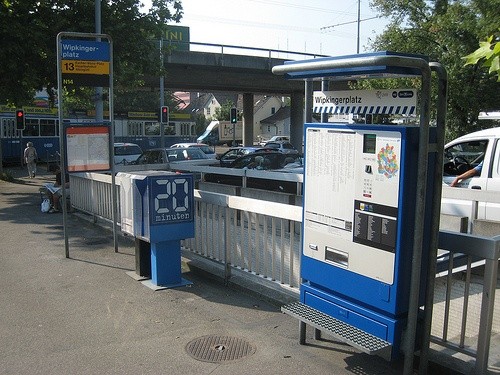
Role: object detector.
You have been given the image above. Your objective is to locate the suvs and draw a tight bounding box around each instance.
[96,143,143,175]
[264,141,299,153]
[170,143,220,160]
[260,136,290,147]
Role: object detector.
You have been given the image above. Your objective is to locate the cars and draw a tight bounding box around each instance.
[215,146,304,196]
[119,147,220,189]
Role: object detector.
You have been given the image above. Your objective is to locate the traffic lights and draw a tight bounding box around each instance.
[161,106,169,124]
[16,110,25,130]
[230,108,237,123]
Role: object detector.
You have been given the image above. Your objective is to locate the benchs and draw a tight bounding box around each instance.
[39,188,70,209]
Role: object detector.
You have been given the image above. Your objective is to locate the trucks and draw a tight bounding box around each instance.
[197,121,244,146]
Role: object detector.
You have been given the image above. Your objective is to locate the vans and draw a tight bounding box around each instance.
[442,127,500,203]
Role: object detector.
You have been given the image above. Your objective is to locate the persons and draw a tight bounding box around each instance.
[450,159,484,187]
[45,162,70,213]
[24,142,37,179]
